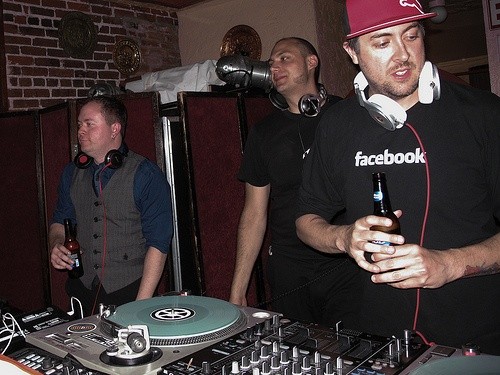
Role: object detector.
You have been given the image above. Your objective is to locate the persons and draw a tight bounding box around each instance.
[48,95,173,320]
[226,36,350,328]
[295,0,500,356]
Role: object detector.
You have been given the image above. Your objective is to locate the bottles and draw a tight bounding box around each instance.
[368,172,405,274]
[63,217,84,279]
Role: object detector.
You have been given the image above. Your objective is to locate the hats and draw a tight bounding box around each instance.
[343,0,437,40]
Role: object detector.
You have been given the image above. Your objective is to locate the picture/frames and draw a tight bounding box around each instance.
[487,0,500,30]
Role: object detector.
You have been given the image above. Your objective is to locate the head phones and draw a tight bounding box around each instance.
[353,59,441,132]
[269,83,328,116]
[74,141,129,170]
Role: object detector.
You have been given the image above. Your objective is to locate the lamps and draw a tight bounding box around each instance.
[215,56,274,94]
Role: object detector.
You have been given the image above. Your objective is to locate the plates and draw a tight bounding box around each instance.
[112,39,141,75]
[219,25,262,60]
[58,13,96,60]
